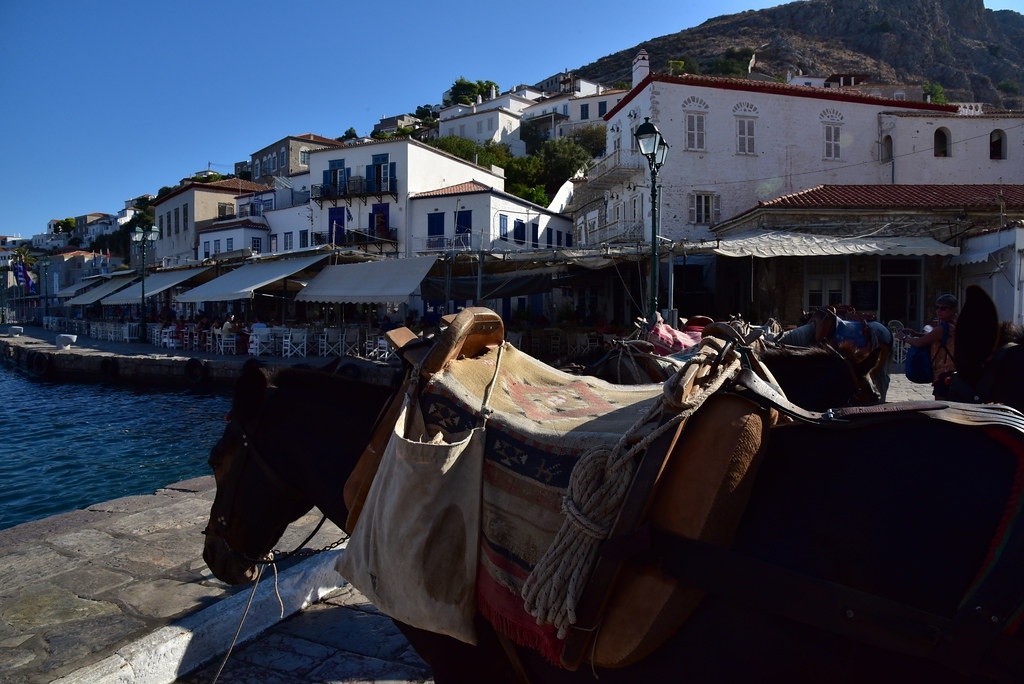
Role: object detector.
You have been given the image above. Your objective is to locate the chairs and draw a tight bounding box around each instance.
[888,320,904,332]
[506,329,629,354]
[42,316,400,362]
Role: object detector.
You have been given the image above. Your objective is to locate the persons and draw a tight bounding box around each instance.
[251,316,267,331]
[381,315,397,336]
[895,294,967,401]
[371,314,380,328]
[189,309,204,323]
[175,315,185,333]
[196,317,222,341]
[406,316,416,329]
[221,316,240,355]
[420,316,432,329]
[162,317,177,329]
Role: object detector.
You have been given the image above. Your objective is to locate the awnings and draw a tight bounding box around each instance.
[712,228,961,258]
[175,253,331,302]
[56,279,102,297]
[295,256,438,304]
[67,276,138,304]
[951,245,1007,265]
[100,265,213,305]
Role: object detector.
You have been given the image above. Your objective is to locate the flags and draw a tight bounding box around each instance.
[12,261,36,293]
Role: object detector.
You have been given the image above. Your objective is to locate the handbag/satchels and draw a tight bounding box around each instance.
[333,339,502,647]
[905,344,933,383]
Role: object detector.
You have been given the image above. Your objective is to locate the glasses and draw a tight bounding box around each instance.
[936,306,948,311]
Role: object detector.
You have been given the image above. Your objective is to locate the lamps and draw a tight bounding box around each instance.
[626,181,636,191]
[627,110,637,120]
[610,124,619,133]
[610,191,618,200]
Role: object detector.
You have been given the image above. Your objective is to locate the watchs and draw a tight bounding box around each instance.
[903,335,907,340]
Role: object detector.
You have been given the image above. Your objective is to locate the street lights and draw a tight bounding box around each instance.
[129,224,160,343]
[633,117,670,316]
[41,255,52,316]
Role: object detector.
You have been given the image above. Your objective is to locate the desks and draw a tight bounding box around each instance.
[245,331,274,355]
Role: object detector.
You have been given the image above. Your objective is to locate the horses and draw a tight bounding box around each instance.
[932,285,1024,417]
[200,355,1024,683]
[583,306,896,420]
